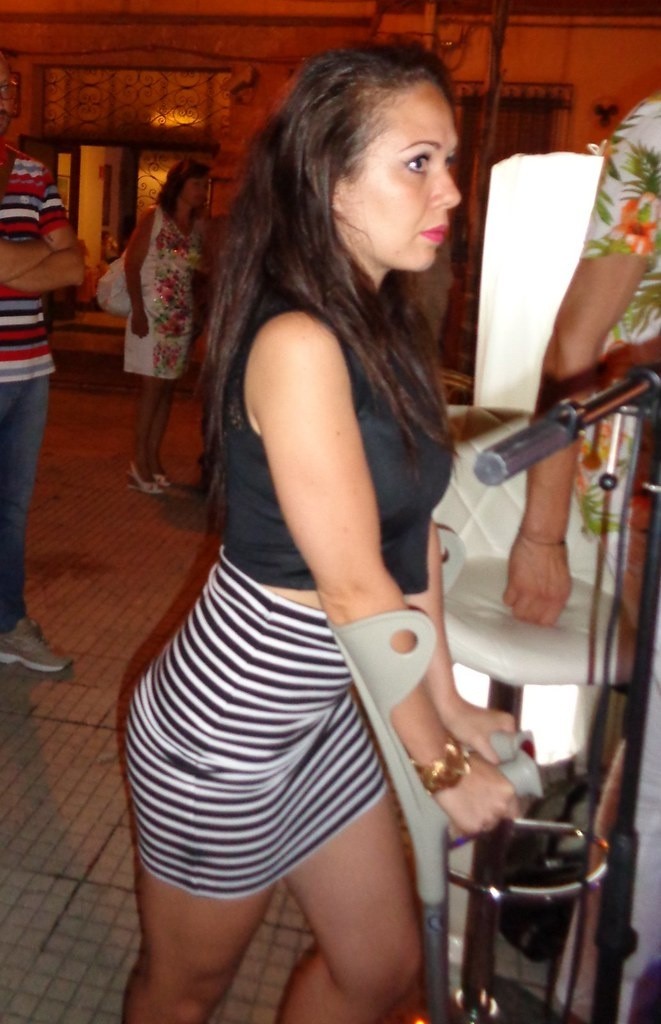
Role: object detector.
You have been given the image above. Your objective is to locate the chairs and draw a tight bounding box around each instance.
[433,152,661,1024]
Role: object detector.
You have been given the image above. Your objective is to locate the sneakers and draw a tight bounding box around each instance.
[0,617,72,672]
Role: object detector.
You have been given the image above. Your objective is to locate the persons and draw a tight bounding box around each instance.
[500,98,661,629]
[0,50,83,675]
[120,42,521,1024]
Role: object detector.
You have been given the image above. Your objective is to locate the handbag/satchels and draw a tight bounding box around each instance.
[96,204,163,318]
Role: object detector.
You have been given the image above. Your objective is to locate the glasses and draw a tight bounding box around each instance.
[0,84,17,100]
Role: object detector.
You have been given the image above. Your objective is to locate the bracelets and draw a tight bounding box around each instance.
[519,527,567,546]
[408,733,473,795]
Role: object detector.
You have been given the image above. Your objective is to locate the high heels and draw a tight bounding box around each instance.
[151,468,171,488]
[126,461,165,495]
